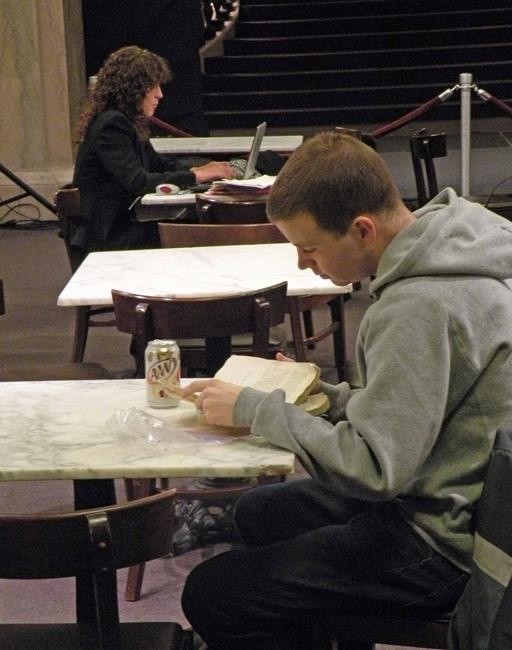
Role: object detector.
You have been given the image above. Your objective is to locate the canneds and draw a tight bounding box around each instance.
[143,338,182,407]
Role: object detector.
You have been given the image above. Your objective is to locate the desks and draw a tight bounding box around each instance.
[148,135,303,161]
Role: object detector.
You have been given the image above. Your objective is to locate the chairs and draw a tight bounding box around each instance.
[410,125,446,207]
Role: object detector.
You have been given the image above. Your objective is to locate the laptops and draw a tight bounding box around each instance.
[188,121,267,193]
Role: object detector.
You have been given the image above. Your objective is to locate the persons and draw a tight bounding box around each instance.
[67,44,242,254]
[172,130,512,649]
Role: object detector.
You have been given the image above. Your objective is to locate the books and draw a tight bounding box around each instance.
[139,174,277,205]
[165,352,332,426]
